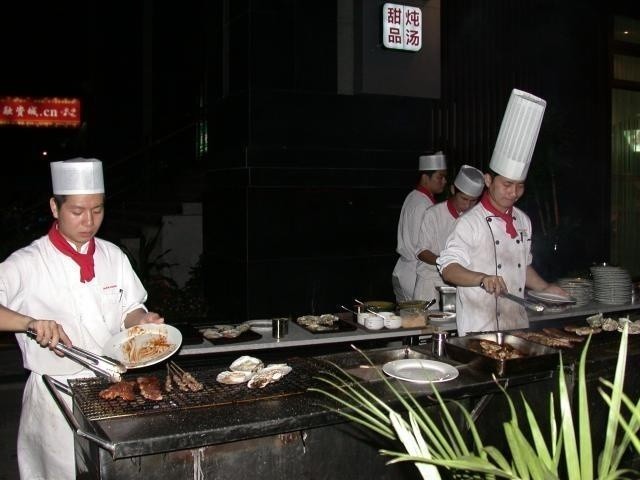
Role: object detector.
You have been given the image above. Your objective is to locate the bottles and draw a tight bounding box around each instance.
[433,330,449,358]
[271,319,284,338]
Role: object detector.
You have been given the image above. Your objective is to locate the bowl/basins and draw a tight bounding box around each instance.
[363,301,393,312]
[356,312,403,331]
[400,308,428,329]
[399,301,429,310]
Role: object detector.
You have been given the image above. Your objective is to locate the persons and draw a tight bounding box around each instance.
[0,156,169,479]
[436,167,571,337]
[412,165,487,303]
[391,149,448,304]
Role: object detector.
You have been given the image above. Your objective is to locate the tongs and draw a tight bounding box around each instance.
[25,328,126,384]
[480,282,545,313]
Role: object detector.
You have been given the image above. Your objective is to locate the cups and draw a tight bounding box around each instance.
[281,318,289,334]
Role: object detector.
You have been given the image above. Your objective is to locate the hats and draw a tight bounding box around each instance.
[453,163,485,198]
[49,155,104,197]
[491,88,549,181]
[417,152,447,173]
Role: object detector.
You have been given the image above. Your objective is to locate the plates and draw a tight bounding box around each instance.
[431,312,457,321]
[245,318,274,331]
[383,358,459,384]
[100,322,184,369]
[528,290,576,305]
[556,264,630,307]
